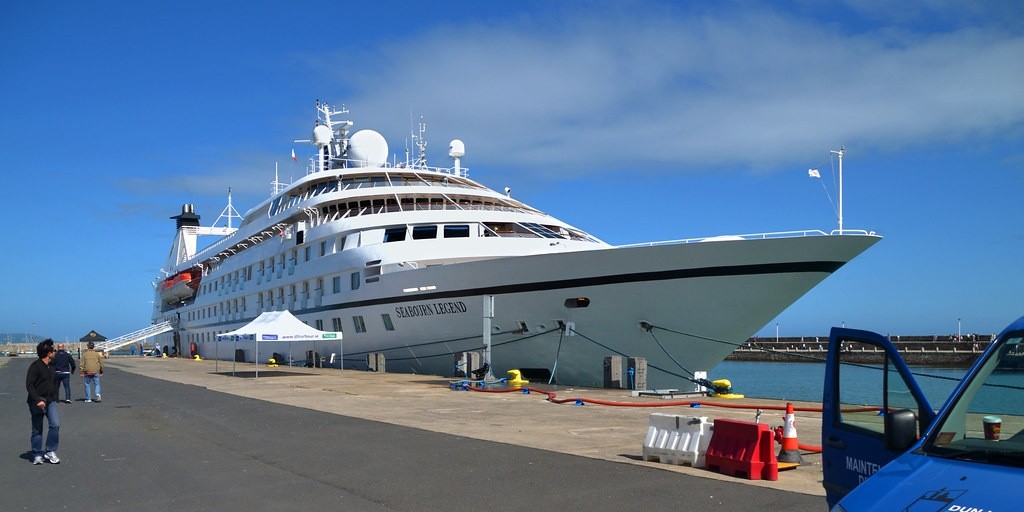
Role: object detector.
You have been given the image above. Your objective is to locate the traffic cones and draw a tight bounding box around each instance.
[774,402,804,470]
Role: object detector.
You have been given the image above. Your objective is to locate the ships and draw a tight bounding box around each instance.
[151,96,886,390]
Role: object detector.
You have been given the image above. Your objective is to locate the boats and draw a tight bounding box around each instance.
[171,273,196,300]
[161,280,181,305]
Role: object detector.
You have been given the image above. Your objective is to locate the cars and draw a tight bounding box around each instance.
[821,312,1024,512]
[143,346,153,355]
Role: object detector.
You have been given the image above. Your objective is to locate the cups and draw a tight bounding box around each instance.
[983,416,1002,441]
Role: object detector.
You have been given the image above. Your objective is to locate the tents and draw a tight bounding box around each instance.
[217,310,342,378]
[80,330,108,360]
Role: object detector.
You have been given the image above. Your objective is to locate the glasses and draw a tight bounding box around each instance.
[53,349,56,352]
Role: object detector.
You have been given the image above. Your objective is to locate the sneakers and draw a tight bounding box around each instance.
[33,456,43,465]
[85,399,91,402]
[95,394,101,401]
[44,451,60,464]
[66,399,72,404]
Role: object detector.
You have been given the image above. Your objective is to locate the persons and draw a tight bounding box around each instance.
[79,342,106,403]
[24,338,62,466]
[129,343,144,356]
[52,344,76,405]
[152,343,161,360]
